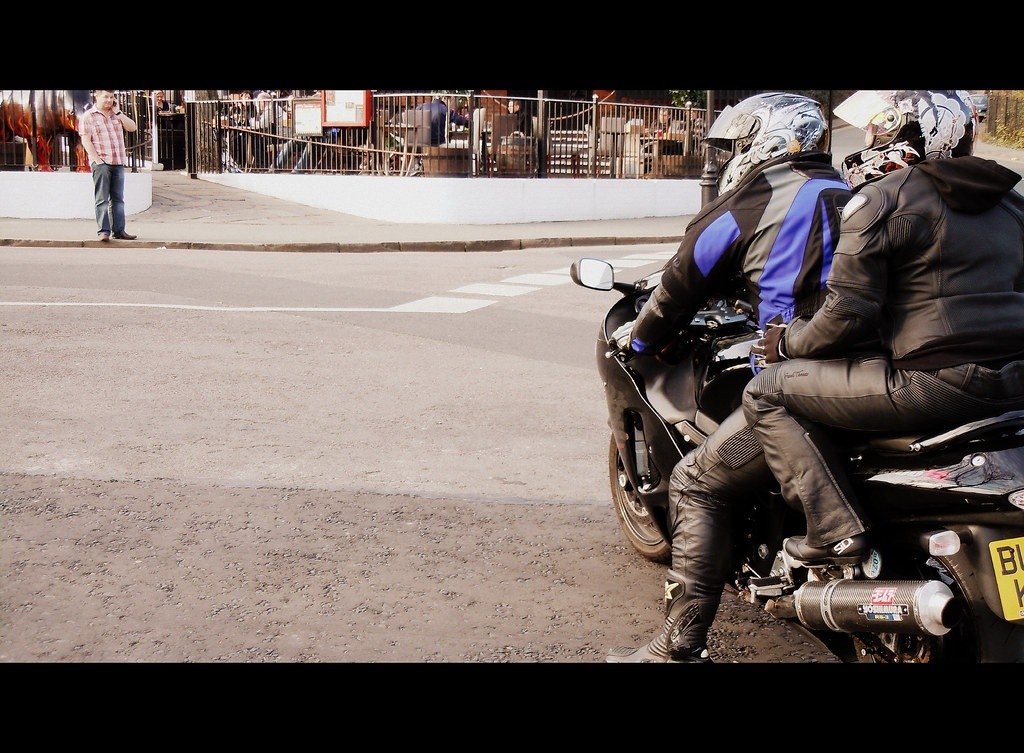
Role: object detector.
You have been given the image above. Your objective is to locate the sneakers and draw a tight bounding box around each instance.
[782,531,870,566]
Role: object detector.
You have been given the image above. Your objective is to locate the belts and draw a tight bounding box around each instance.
[937,369,1023,399]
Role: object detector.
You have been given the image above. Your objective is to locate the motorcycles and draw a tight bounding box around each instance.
[569,251,1024,665]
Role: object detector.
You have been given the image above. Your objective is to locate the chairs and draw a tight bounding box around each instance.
[402,109,431,173]
[485,114,518,178]
[596,117,626,178]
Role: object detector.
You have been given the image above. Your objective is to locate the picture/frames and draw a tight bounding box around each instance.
[291,99,323,137]
[321,90,369,127]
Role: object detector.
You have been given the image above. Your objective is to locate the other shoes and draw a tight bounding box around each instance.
[409,165,421,176]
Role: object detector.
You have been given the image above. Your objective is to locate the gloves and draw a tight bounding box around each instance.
[751,325,788,366]
[610,319,638,350]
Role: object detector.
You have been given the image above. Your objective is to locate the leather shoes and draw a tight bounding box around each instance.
[98,234,109,241]
[112,231,137,240]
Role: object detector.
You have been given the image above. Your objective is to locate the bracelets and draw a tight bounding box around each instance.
[115,110,123,116]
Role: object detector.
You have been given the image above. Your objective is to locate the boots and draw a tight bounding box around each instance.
[606,574,720,663]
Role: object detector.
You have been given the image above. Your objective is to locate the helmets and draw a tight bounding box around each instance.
[705,91,828,198]
[842,91,980,192]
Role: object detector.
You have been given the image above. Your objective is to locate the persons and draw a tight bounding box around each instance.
[409,90,474,148]
[506,99,534,145]
[741,90,1024,571]
[687,110,708,141]
[149,90,169,111]
[268,125,342,175]
[248,93,289,169]
[606,89,883,660]
[76,90,138,242]
[651,108,672,132]
[229,89,268,170]
